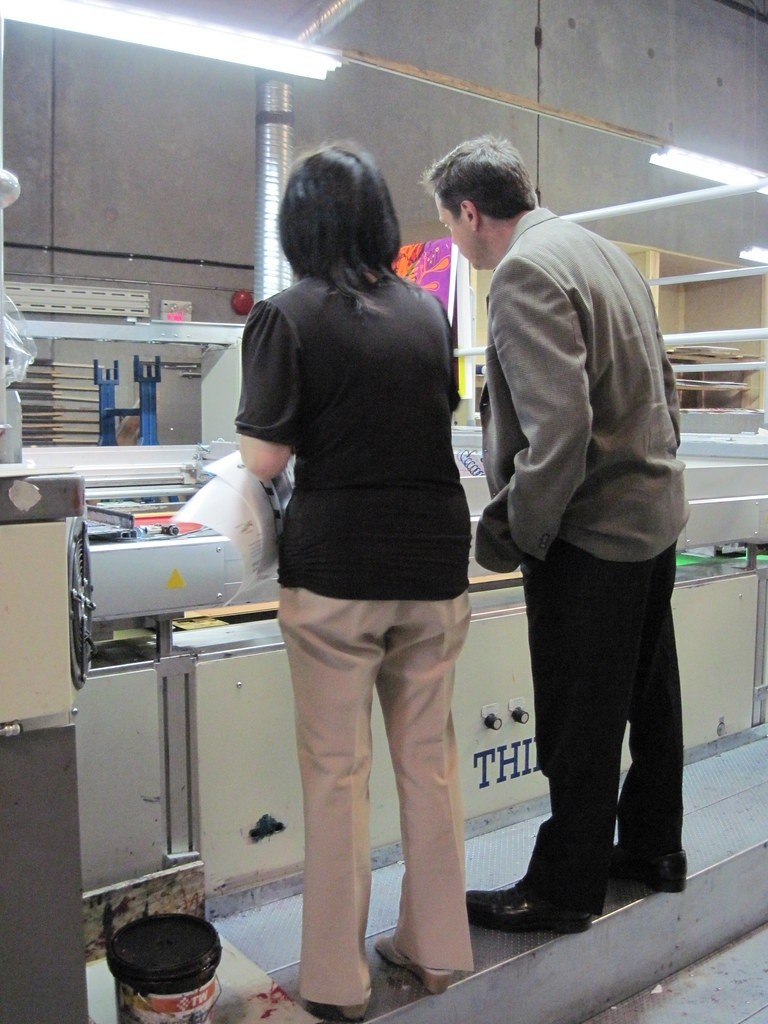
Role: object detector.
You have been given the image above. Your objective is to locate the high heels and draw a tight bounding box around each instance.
[336,998,370,1022]
[375,935,453,994]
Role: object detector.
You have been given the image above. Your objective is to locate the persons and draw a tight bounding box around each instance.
[419,132,692,935]
[233,146,475,1024]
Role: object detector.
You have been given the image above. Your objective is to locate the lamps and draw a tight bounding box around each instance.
[0,0,347,79]
[649,145,768,197]
[738,243,768,264]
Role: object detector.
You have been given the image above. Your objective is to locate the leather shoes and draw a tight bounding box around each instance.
[466,879,592,934]
[609,843,687,893]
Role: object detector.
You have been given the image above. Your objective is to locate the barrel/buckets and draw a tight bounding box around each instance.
[106,913,221,1024]
